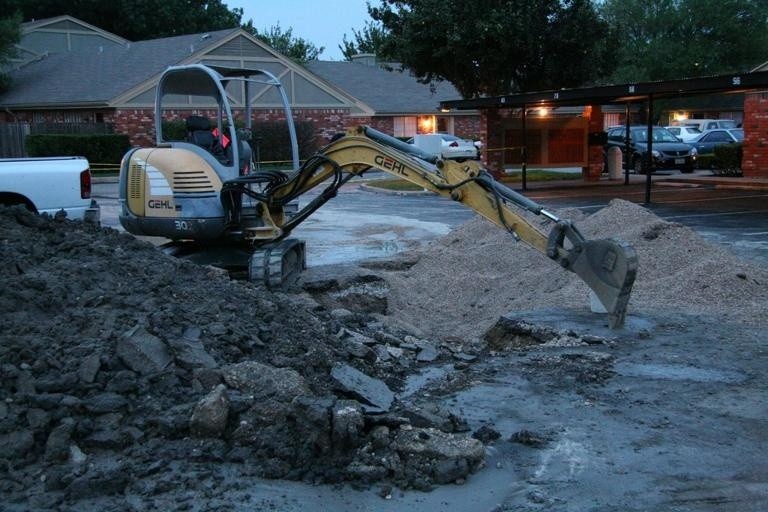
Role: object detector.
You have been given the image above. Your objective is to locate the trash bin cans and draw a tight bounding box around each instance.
[608,146,622,181]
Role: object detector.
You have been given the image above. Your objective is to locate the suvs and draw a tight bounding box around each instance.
[602,124,698,174]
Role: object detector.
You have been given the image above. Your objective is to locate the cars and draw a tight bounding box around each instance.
[402,130,478,163]
[643,117,743,170]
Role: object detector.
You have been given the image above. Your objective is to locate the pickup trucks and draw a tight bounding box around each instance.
[0,153,102,226]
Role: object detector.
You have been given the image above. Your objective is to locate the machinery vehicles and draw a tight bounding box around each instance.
[113,60,639,337]
